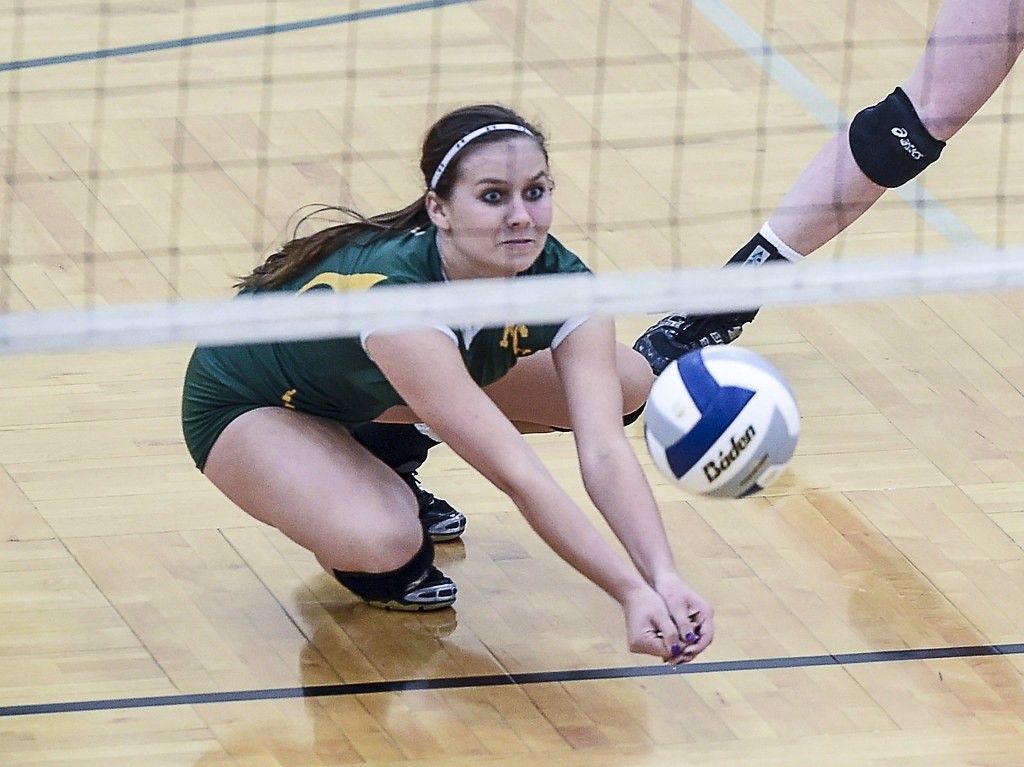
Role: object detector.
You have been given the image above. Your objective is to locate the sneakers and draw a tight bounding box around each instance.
[361,563,457,611]
[632,310,743,376]
[402,470,467,542]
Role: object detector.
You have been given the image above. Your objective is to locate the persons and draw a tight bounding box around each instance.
[181,105,715,666]
[629,1,1024,379]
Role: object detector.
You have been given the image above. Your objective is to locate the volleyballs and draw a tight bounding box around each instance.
[644,344,801,499]
